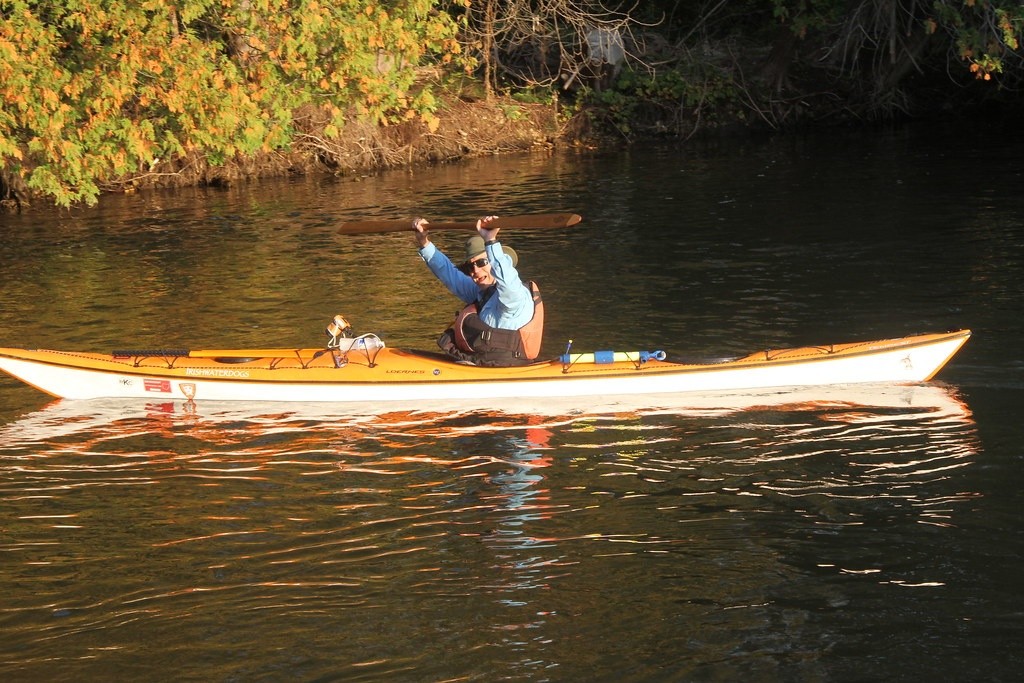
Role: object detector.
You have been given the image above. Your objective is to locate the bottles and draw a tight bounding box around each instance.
[339,337,384,351]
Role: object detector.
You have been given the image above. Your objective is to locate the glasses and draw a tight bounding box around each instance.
[466,258,489,273]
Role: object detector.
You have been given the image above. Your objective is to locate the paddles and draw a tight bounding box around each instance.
[329,210,582,236]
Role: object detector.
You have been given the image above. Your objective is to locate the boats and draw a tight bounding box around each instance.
[0,326,974,403]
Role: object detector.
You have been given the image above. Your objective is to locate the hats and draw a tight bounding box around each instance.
[460,237,518,268]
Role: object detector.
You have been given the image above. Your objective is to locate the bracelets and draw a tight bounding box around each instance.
[485,240,499,247]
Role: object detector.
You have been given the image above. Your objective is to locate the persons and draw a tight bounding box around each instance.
[412,215,544,367]
